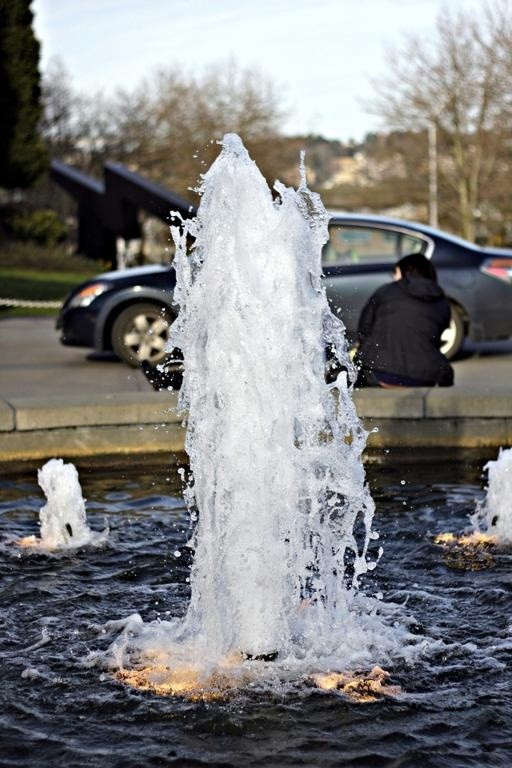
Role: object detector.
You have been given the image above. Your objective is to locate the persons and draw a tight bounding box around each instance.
[351,253,454,386]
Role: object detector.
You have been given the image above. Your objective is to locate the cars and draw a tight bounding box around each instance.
[55,212,512,374]
